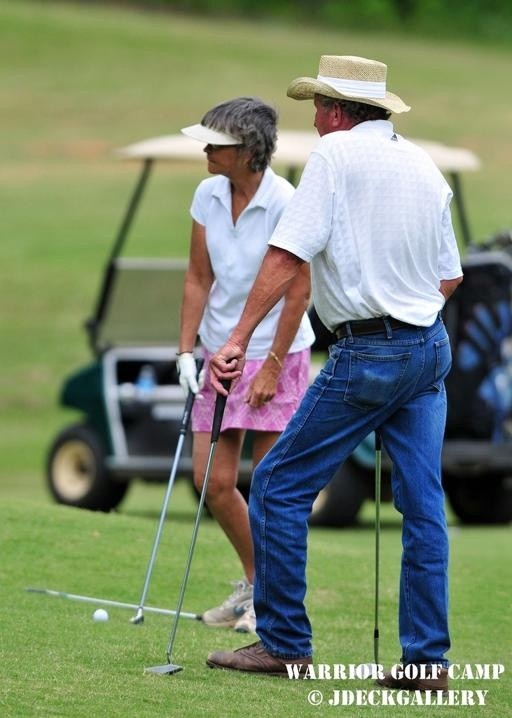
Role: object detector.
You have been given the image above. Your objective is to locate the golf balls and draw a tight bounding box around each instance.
[93,608,109,622]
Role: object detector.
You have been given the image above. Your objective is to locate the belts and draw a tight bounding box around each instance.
[336,316,409,339]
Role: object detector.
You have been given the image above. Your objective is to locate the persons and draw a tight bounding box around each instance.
[206,51,468,698]
[173,96,320,636]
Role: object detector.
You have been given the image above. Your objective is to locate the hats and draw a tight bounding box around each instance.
[181,123,243,145]
[287,56,410,113]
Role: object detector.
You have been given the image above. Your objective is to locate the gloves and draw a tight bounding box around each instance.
[176,351,199,394]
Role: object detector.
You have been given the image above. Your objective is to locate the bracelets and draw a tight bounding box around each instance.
[269,351,284,369]
[175,350,194,355]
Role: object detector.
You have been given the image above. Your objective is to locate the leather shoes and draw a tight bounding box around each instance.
[206,641,312,679]
[376,667,448,697]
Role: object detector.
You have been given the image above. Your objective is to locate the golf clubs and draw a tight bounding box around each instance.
[357,430,384,675]
[143,359,235,673]
[131,359,205,624]
[25,588,202,620]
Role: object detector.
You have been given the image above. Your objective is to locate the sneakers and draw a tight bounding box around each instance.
[203,580,257,632]
